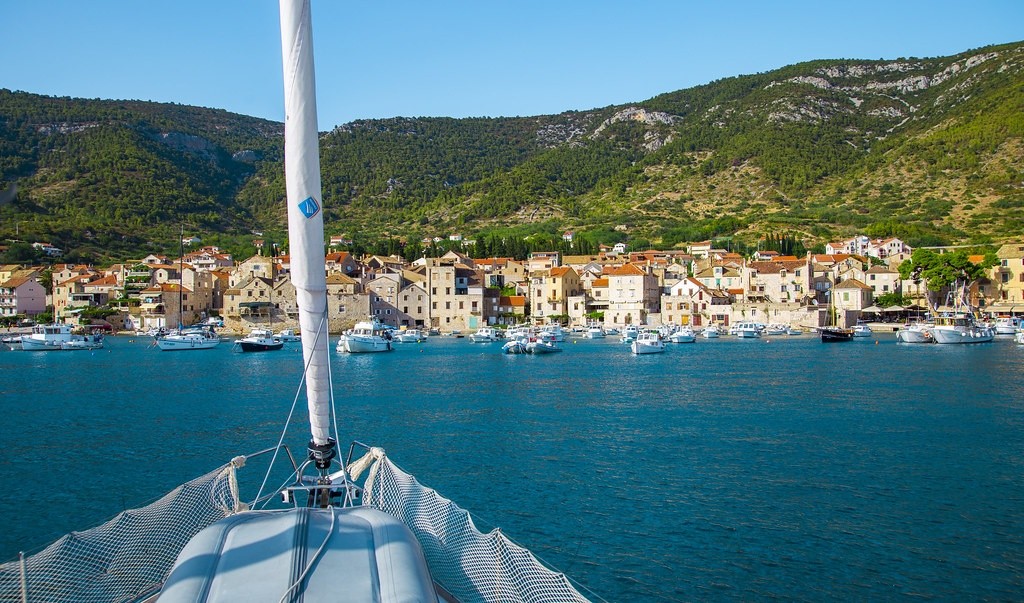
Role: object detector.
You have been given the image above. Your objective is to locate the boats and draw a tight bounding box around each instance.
[0,305,1024,356]
[0,434,615,603]
